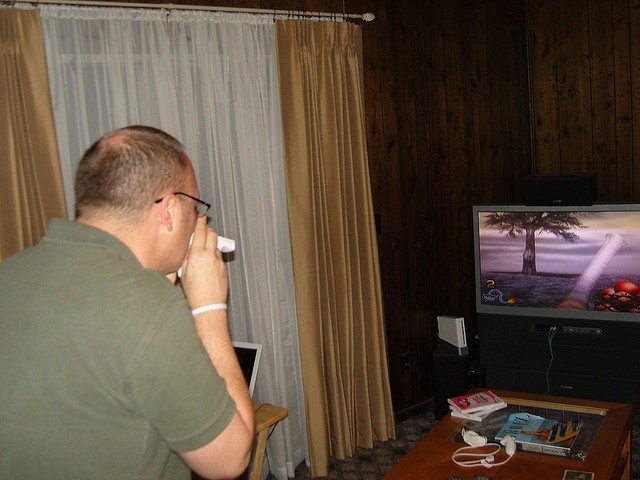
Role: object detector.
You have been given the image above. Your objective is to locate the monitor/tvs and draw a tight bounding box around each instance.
[472,203,640,323]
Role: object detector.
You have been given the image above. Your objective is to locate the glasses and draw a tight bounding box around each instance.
[154,192,212,217]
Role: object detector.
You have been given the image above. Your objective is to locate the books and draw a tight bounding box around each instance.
[494,413,584,458]
[447,390,507,422]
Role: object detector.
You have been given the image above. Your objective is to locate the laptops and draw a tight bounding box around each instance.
[231,341,263,399]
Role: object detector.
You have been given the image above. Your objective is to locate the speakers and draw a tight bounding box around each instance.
[433,343,470,420]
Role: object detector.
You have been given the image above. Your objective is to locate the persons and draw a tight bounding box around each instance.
[1,125,256,479]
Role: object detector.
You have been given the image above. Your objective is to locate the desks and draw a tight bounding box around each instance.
[248,401,288,479]
[379,388,633,479]
